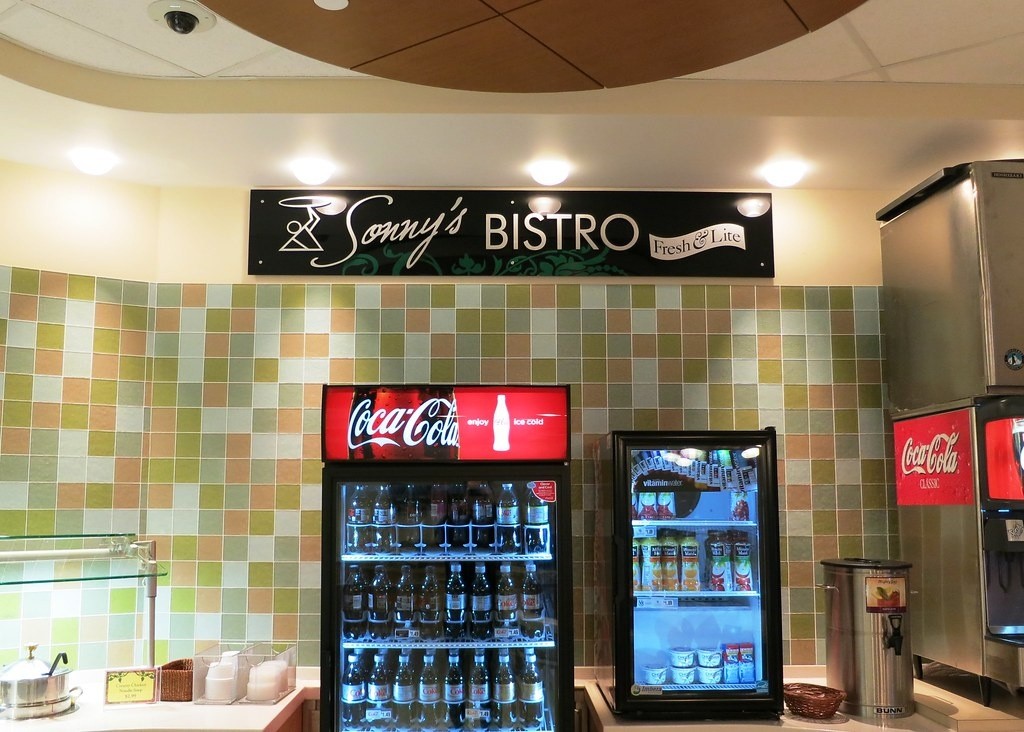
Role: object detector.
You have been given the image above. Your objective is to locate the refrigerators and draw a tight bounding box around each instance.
[320,382,575,731]
[593,427,784,719]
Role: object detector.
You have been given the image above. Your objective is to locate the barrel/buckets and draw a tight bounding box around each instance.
[820,558,916,720]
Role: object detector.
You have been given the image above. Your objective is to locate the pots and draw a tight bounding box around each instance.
[0,644,83,717]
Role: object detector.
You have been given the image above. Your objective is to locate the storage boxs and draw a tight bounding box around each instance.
[192,641,299,705]
[721,642,755,683]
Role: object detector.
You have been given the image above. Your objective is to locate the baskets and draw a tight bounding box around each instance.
[160,659,193,701]
[784,683,847,719]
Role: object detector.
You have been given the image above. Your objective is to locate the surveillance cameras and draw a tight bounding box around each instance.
[163,11,198,36]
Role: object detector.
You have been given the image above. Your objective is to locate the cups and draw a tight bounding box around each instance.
[205,651,288,702]
[641,642,722,685]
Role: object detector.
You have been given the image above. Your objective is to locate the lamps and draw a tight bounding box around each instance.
[529,197,561,216]
[312,196,347,216]
[737,198,771,217]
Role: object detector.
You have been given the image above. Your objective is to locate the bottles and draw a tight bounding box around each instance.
[342,485,551,727]
[633,491,754,593]
